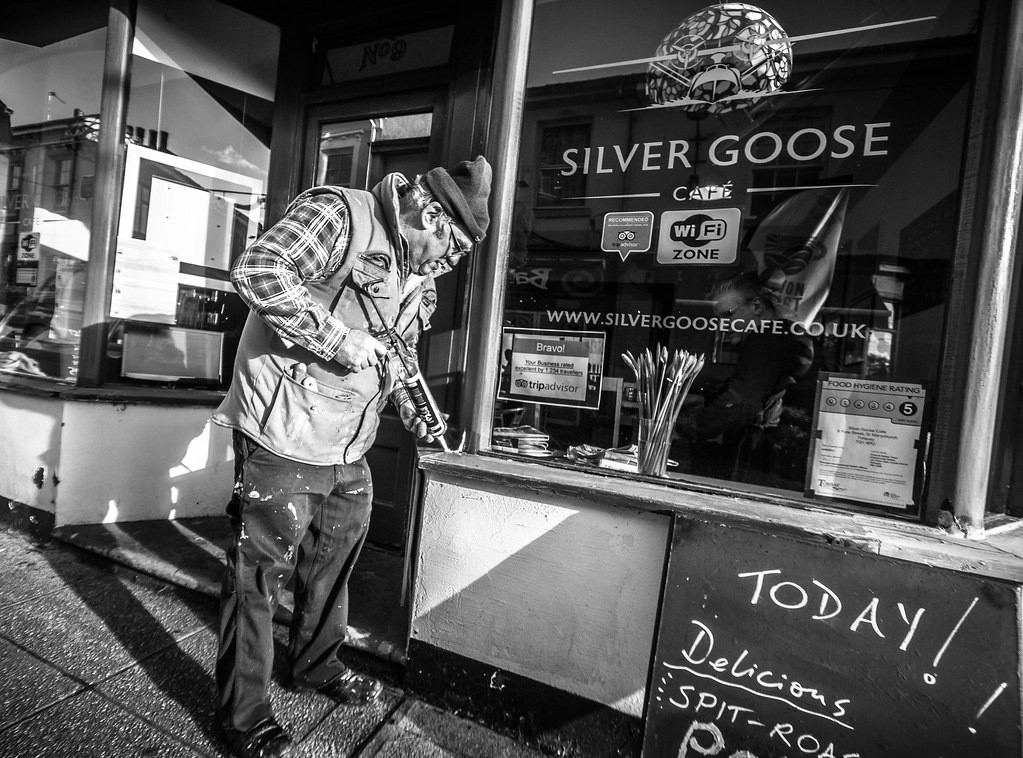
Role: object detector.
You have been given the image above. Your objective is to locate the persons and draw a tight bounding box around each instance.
[210,155,494,758]
[675,271,815,440]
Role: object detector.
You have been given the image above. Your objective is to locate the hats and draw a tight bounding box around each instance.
[427,155,492,245]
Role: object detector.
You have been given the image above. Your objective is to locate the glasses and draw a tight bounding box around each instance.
[715,298,753,323]
[429,199,467,258]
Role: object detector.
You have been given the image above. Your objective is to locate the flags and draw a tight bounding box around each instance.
[743,178,853,327]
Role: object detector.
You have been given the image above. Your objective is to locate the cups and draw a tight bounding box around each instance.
[638,417,676,477]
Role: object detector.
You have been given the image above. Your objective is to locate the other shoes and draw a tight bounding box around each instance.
[211,715,298,758]
[317,667,384,705]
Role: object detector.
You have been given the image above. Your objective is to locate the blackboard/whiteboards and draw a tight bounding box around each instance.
[638,509,1023,758]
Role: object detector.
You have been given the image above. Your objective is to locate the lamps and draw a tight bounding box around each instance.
[646,2,793,117]
